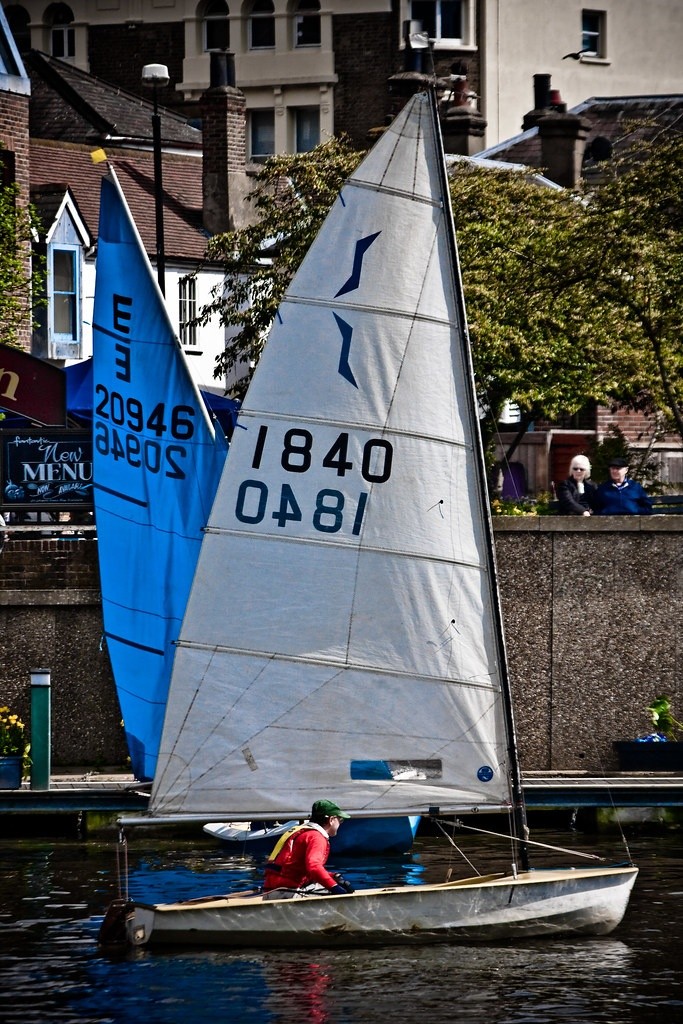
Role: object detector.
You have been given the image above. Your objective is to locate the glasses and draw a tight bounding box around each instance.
[572,467,588,472]
[331,817,344,823]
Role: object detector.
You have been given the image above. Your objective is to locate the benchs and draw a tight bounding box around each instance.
[547,494,683,516]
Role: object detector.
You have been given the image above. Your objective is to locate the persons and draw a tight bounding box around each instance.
[554,454,653,516]
[261,799,356,901]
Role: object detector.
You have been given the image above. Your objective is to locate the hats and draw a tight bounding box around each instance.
[607,455,628,467]
[311,799,351,820]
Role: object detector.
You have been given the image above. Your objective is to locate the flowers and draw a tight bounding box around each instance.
[0,706,26,758]
[646,695,674,742]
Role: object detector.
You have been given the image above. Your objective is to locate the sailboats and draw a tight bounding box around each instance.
[97,38,641,962]
[89,145,430,860]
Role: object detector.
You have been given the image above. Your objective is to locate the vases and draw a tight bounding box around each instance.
[0,756,26,789]
[612,741,683,772]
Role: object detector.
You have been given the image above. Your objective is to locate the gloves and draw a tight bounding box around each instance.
[334,874,355,893]
[330,886,347,895]
